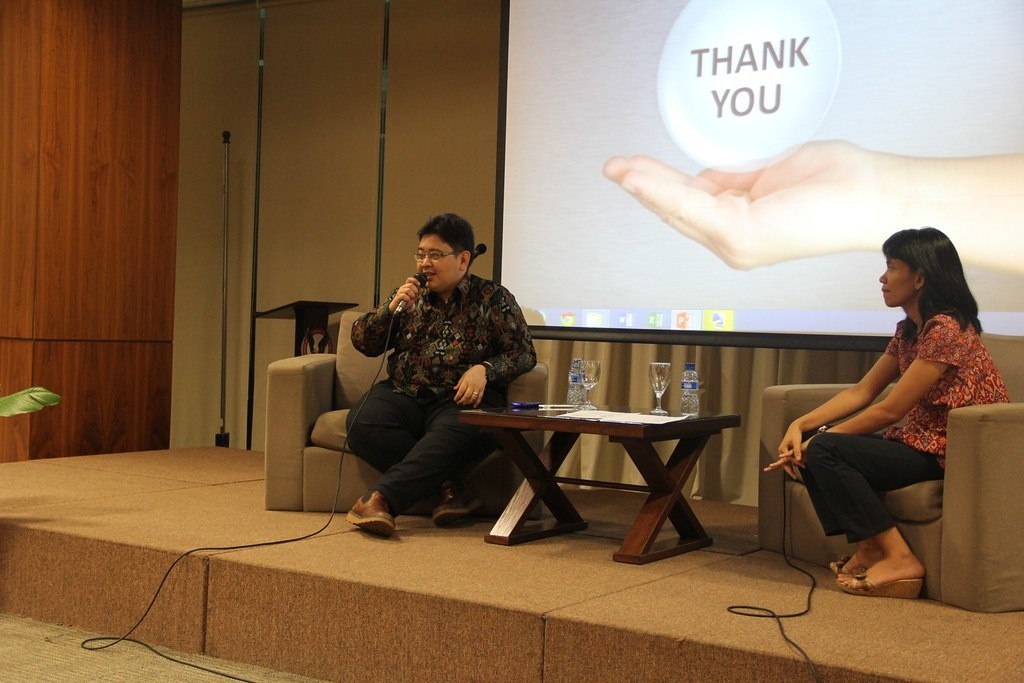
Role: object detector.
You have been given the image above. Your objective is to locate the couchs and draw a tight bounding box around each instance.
[265,310,548,520]
[758,332,1024,615]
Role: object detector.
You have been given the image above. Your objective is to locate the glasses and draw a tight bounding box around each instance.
[414,250,462,260]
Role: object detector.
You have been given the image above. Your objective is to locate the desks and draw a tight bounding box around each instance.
[458,402,742,567]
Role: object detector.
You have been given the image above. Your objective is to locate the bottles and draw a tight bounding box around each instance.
[681,363,700,419]
[567,359,584,405]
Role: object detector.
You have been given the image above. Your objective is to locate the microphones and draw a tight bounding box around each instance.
[471,244,487,260]
[391,272,427,319]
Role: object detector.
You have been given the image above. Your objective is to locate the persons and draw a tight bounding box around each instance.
[601,139,1024,277]
[344,213,538,536]
[764,228,1014,599]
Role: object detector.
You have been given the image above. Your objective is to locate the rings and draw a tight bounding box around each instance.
[473,392,479,395]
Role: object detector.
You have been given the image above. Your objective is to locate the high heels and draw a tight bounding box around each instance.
[832,558,923,600]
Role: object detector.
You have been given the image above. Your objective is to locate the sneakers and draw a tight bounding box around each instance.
[346,489,396,539]
[432,484,482,522]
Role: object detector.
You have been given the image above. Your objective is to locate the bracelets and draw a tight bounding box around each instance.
[817,425,828,435]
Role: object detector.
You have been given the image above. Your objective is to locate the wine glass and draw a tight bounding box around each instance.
[580,361,601,410]
[648,362,671,414]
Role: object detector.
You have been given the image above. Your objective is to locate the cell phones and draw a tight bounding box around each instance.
[511,402,542,409]
